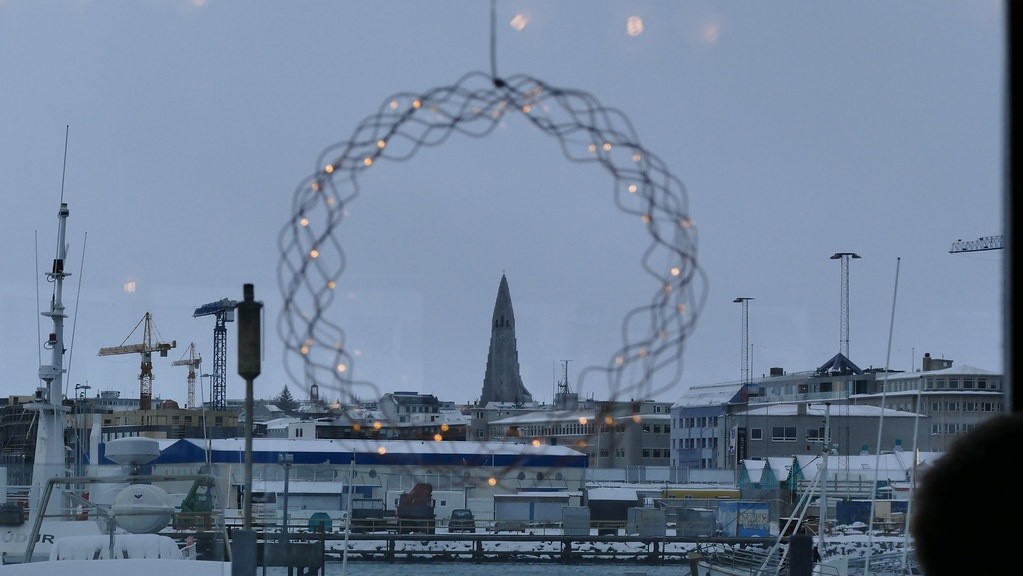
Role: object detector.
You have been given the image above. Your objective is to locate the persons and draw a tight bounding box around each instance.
[913,416,1023,576]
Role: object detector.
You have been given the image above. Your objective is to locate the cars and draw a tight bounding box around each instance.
[448,509,476,533]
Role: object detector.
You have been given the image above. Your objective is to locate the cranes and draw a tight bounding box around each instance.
[95,312,176,410]
[172,342,202,408]
